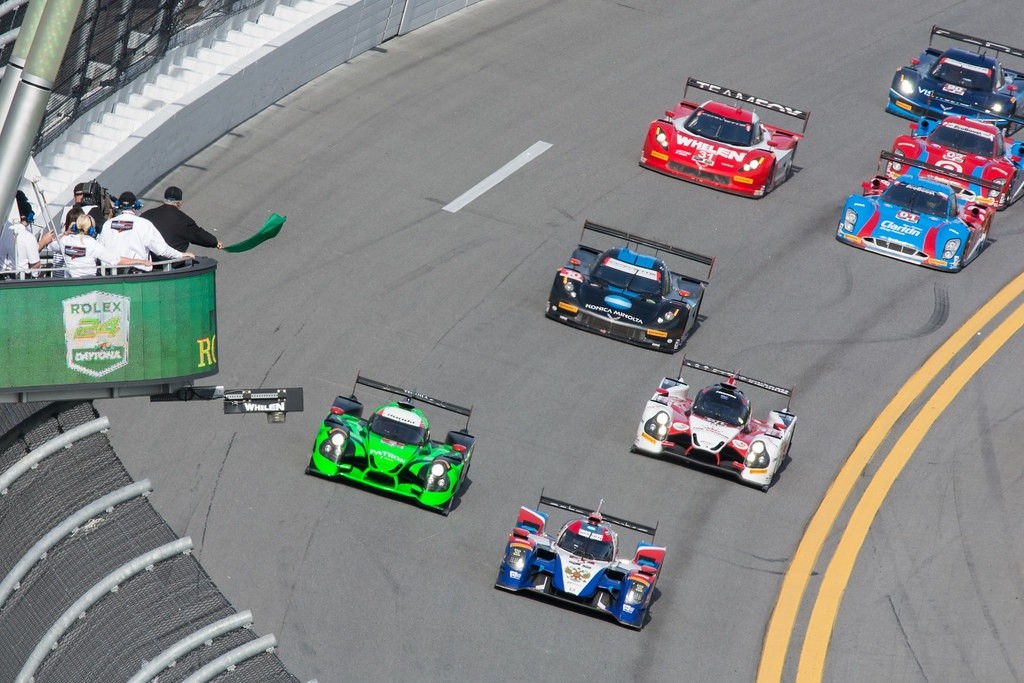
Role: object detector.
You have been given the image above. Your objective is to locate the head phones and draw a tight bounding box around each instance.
[114,199,143,210]
[72,214,97,236]
[27,203,34,223]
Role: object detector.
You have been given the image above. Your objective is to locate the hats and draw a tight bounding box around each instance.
[119,191,136,206]
[165,186,182,200]
[20,202,34,218]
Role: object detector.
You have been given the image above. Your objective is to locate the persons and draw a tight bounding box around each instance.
[16,182,227,277]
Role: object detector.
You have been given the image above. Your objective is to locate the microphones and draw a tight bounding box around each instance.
[108,193,117,203]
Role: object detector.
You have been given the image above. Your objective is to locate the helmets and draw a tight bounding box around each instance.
[588,511,601,521]
